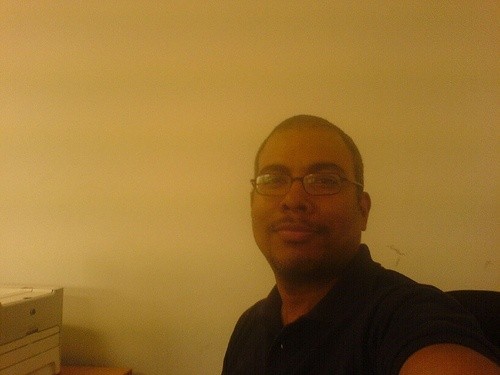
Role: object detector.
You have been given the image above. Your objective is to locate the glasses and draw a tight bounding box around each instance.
[249,171,364,198]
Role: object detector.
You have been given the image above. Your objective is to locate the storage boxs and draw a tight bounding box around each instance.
[0,286,64,375]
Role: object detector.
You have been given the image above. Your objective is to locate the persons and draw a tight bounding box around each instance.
[222,115,499,374]
